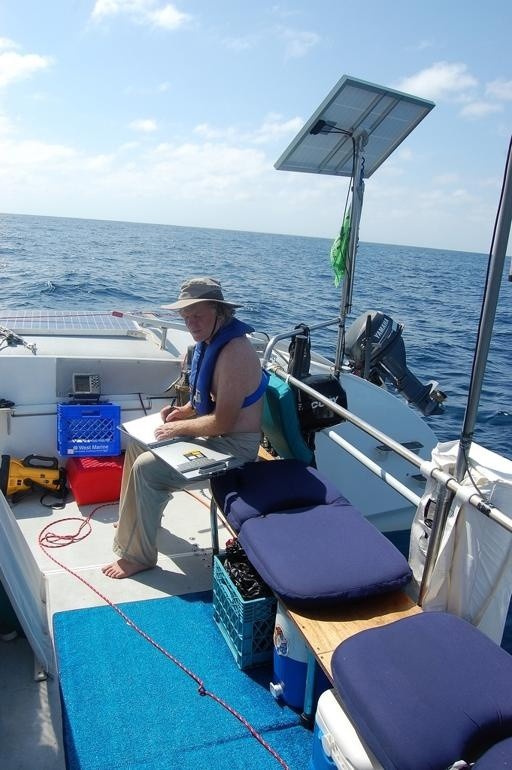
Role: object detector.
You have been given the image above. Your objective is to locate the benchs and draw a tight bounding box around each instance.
[203,438,511,769]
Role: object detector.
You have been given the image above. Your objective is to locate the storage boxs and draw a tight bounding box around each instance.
[210,551,386,769]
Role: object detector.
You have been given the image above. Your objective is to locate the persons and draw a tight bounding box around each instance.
[101,275,266,581]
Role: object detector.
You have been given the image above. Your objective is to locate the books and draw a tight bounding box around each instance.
[115,410,244,482]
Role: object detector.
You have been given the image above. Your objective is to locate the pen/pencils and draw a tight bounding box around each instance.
[164,397,176,424]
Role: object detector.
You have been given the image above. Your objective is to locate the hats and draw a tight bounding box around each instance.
[160,278,243,310]
